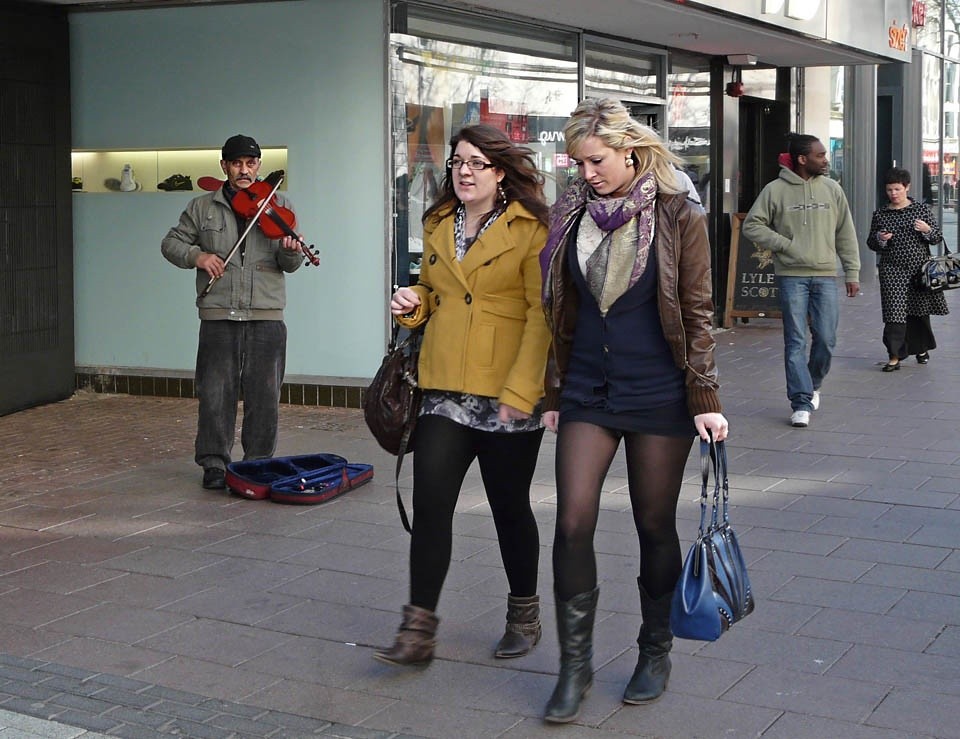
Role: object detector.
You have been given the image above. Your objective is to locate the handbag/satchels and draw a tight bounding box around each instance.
[363,314,420,535]
[922,235,960,292]
[673,428,756,642]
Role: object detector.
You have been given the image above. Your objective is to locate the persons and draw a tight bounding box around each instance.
[866,167,949,372]
[160,134,304,489]
[538,96,728,723]
[742,131,861,428]
[373,123,551,669]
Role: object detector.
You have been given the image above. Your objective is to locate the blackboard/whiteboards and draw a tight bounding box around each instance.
[725,212,784,318]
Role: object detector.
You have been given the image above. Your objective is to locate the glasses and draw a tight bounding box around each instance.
[446,158,494,170]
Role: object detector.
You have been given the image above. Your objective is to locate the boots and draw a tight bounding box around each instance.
[374,604,441,671]
[620,574,681,705]
[493,593,542,658]
[545,587,601,722]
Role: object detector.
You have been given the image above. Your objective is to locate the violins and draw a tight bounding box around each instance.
[231,182,322,267]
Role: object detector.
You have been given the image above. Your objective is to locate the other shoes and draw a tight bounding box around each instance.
[882,362,900,372]
[157,174,193,191]
[203,467,225,489]
[916,352,929,364]
[104,163,143,192]
[790,411,810,427]
[72,177,83,192]
[810,391,821,410]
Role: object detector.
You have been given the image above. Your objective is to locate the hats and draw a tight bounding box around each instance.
[222,134,261,160]
